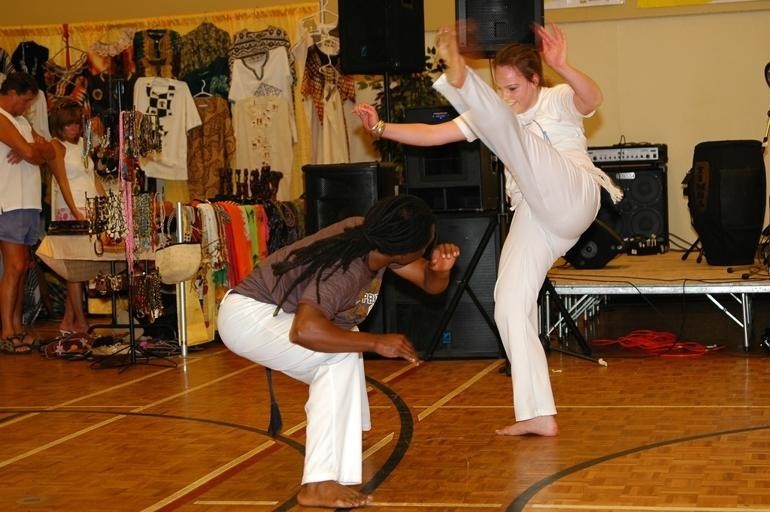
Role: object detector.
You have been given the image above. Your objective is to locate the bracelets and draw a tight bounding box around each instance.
[369,120,386,140]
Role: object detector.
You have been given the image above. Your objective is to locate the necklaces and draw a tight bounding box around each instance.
[81,111,166,324]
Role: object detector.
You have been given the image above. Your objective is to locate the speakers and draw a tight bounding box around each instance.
[562,218,626,269]
[455,0,545,59]
[405,105,499,213]
[338,0,426,74]
[384,210,509,360]
[597,164,668,253]
[301,160,384,359]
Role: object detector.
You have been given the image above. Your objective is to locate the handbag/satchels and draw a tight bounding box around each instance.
[48,219,89,235]
[155,244,202,284]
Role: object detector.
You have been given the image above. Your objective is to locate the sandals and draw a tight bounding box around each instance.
[0,331,43,354]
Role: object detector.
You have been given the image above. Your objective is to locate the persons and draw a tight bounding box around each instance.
[350,17,607,441]
[0,70,58,355]
[214,191,463,509]
[47,98,110,336]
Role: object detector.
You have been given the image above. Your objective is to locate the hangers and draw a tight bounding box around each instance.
[8,0,352,100]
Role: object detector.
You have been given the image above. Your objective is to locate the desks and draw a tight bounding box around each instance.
[34,235,186,359]
